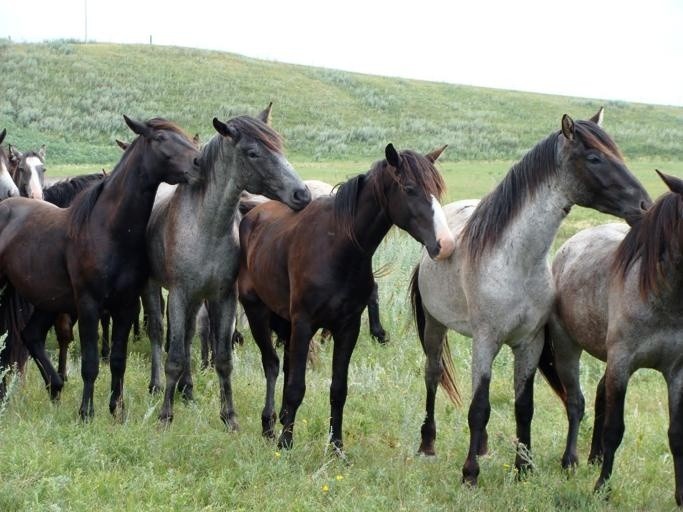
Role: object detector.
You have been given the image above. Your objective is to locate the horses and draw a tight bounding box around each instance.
[408,105,655,493]
[539,168,683,512]
[0,100,455,459]
